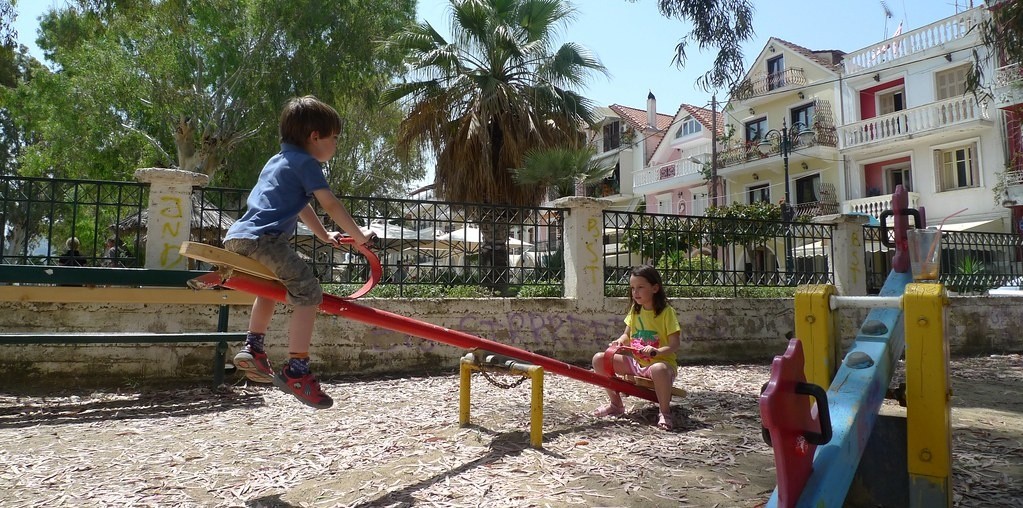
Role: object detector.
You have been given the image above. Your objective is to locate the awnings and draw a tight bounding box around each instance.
[585,116,619,129]
[584,157,619,184]
[602,197,640,236]
[793,218,1004,258]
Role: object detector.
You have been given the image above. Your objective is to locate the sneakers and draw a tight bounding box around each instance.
[233,344,275,383]
[273,365,333,409]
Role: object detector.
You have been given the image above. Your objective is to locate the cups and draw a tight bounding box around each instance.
[907,228,942,283]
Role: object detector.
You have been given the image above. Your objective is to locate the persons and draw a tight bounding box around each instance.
[223,96,377,407]
[592,265,681,431]
[106,236,134,288]
[58,237,87,287]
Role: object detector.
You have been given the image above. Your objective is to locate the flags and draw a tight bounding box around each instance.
[869,21,902,61]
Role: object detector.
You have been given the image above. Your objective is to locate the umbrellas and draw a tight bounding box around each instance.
[340,222,433,263]
[109,191,237,237]
[440,226,531,274]
[403,226,462,279]
[293,221,333,251]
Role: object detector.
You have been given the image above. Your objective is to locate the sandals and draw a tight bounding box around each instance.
[592,402,625,416]
[658,413,673,430]
